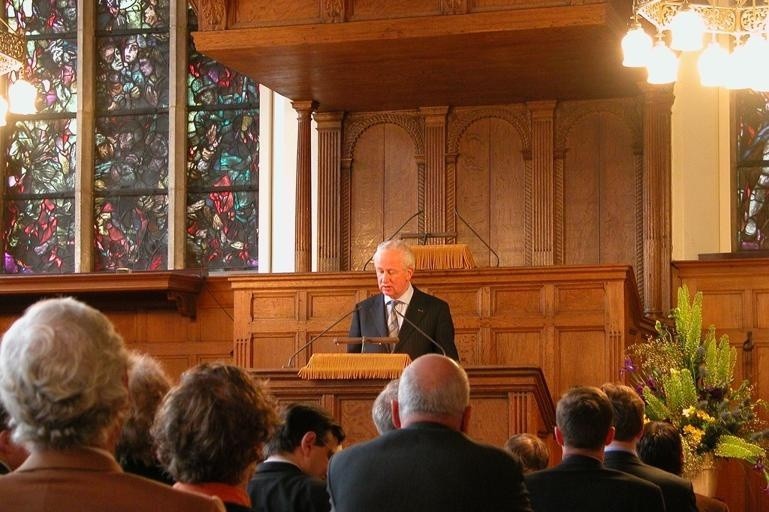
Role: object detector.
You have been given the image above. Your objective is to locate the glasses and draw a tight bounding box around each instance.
[315,435,334,461]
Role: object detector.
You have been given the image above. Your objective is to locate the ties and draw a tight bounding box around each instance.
[385,299,401,353]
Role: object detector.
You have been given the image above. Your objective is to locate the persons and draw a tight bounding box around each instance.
[599,381,698,512]
[325,353,535,512]
[501,431,549,472]
[526,384,668,512]
[117,346,175,485]
[247,401,345,512]
[636,417,733,512]
[347,241,458,366]
[1,294,228,512]
[371,380,401,436]
[151,358,283,512]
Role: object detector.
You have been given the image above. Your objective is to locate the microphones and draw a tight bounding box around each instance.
[453,207,500,267]
[288,305,363,368]
[391,300,447,356]
[362,209,423,271]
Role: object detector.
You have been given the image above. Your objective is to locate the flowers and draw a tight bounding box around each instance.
[626,278,769,487]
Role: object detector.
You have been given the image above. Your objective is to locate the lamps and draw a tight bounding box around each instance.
[620,0,769,94]
[0,16,39,128]
